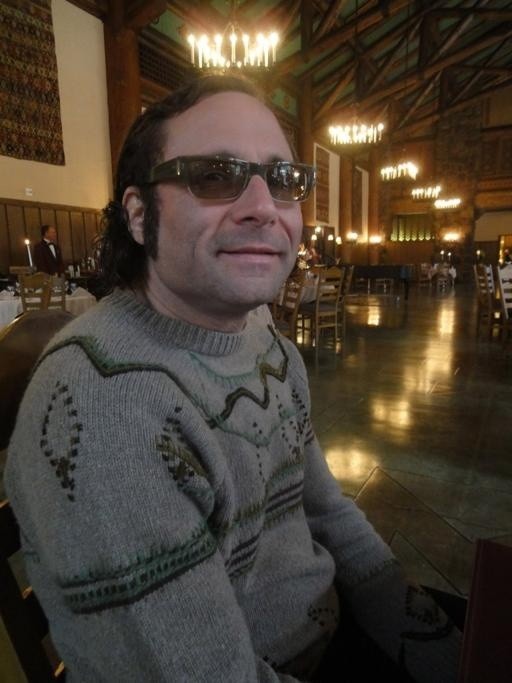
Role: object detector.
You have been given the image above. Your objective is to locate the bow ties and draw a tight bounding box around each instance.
[47,242,55,246]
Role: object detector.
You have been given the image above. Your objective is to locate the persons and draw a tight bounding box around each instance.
[2,72,469,683]
[33,225,64,275]
[500,247,512,269]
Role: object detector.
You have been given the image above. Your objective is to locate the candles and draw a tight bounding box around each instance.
[25,238,33,266]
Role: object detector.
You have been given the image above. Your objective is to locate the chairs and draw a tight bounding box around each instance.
[472,262,511,349]
[0,265,77,683]
[421,262,454,295]
[268,264,354,350]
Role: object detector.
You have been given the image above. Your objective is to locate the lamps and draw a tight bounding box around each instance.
[188,31,278,68]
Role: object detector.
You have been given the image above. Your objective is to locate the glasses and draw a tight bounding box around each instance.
[125,155,316,203]
